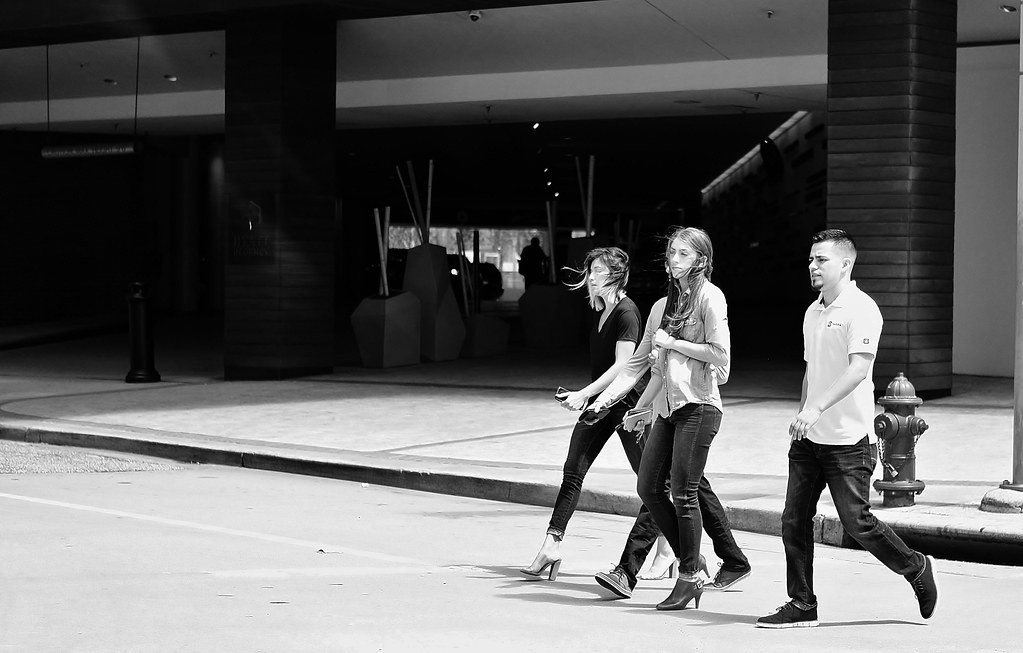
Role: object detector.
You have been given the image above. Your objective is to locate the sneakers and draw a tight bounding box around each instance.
[702,566,752,589]
[757,602,819,628]
[595,566,633,599]
[911,555,939,619]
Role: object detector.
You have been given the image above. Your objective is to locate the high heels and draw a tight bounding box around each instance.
[519,550,563,580]
[656,578,704,611]
[638,548,677,581]
[698,553,709,576]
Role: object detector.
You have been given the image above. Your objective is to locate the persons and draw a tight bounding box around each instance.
[517,247,679,580]
[620,225,732,610]
[757,229,940,627]
[584,257,754,599]
[520,237,550,291]
